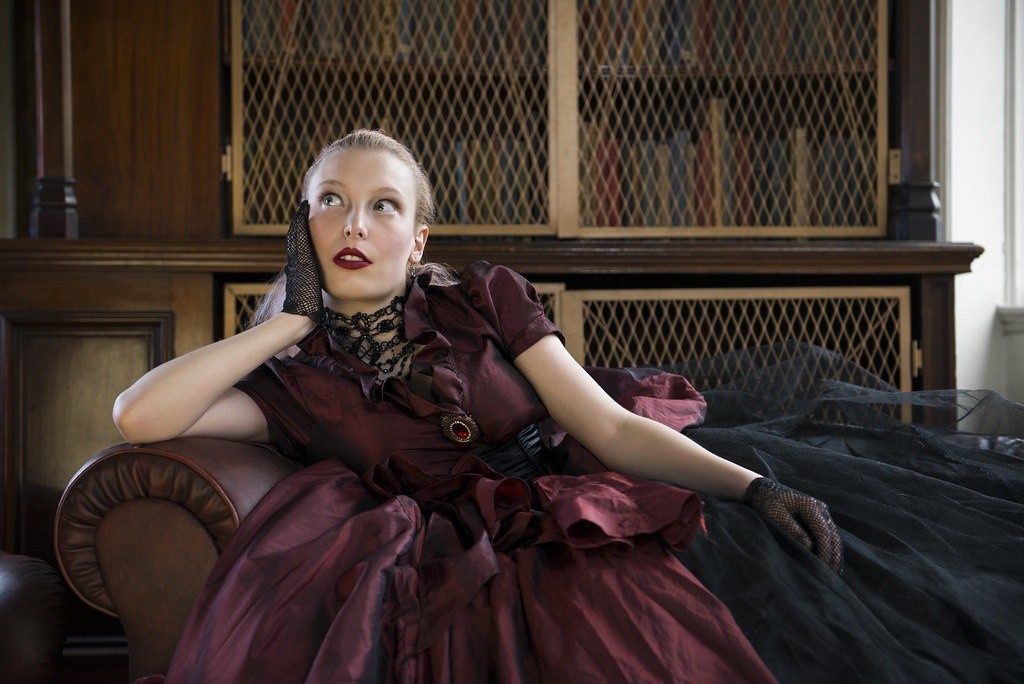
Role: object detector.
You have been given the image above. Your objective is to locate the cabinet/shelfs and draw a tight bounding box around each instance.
[0,2,984,568]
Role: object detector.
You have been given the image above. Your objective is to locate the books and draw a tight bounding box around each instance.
[234,0,886,238]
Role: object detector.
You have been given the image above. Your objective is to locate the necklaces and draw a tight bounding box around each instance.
[382,352,481,445]
[324,300,415,386]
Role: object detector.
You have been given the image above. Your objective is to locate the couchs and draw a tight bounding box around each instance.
[56,431,1023,684]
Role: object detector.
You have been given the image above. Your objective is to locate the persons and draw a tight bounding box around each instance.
[112,128,841,684]
